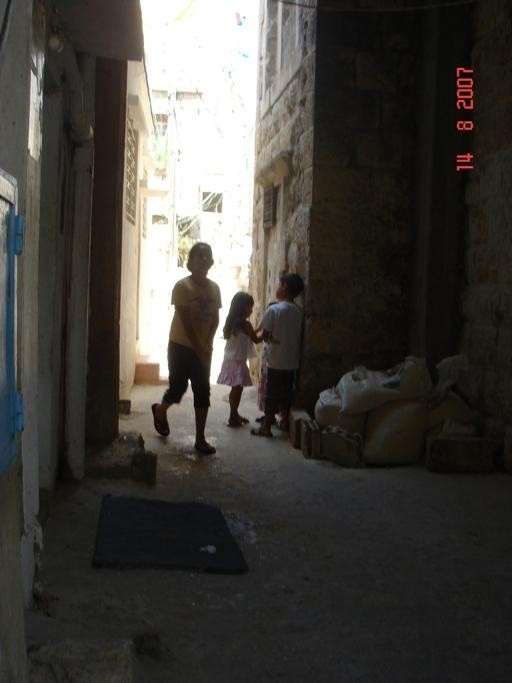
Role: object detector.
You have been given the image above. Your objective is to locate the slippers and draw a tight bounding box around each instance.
[152,403,275,454]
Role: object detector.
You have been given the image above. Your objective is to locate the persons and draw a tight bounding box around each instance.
[217,292,264,426]
[152,243,216,453]
[250,273,303,437]
